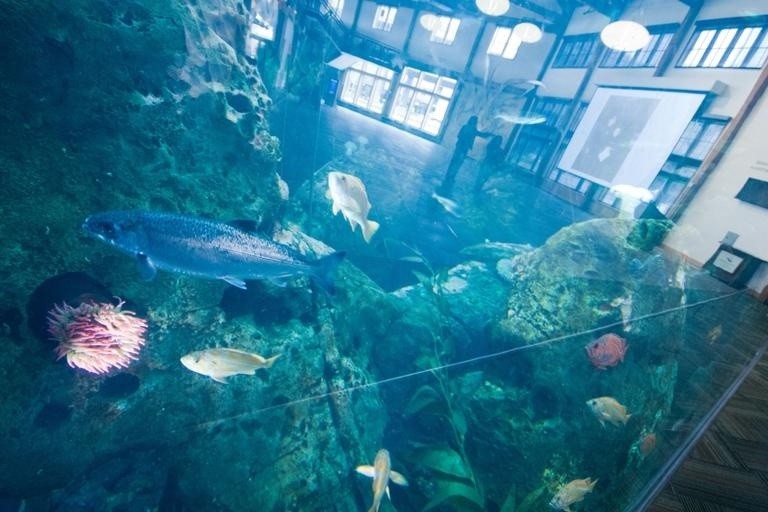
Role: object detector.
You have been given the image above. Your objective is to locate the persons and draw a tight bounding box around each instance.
[443,116,493,193]
[472,136,508,199]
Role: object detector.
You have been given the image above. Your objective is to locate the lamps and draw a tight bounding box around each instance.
[600,1,650,52]
